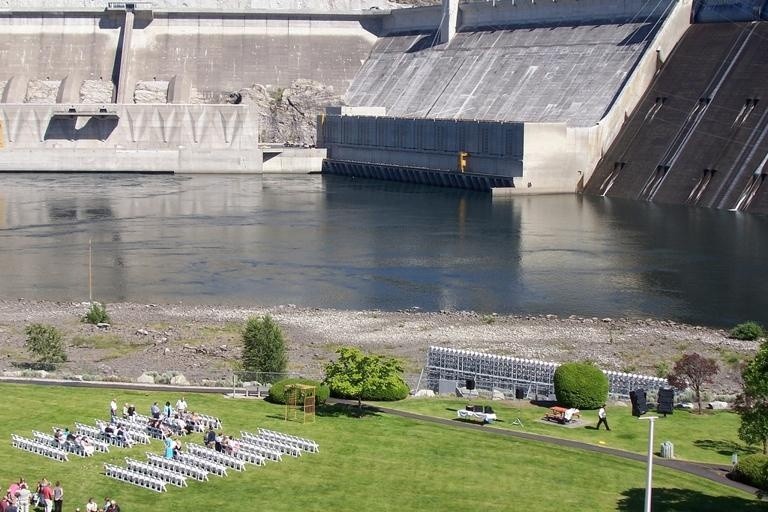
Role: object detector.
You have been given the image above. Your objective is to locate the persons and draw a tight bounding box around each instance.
[76,496,120,512]
[0,477,64,512]
[110,398,118,416]
[164,434,182,459]
[597,404,610,430]
[151,397,188,419]
[204,427,236,453]
[122,403,135,419]
[55,426,89,450]
[105,422,126,440]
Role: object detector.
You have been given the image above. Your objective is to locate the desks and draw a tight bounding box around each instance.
[550,406,580,418]
[457,409,497,423]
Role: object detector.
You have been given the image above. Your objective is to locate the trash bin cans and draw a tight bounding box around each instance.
[660,441,673,458]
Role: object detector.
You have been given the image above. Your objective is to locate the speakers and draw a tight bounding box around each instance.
[516,388,523,399]
[465,378,475,389]
